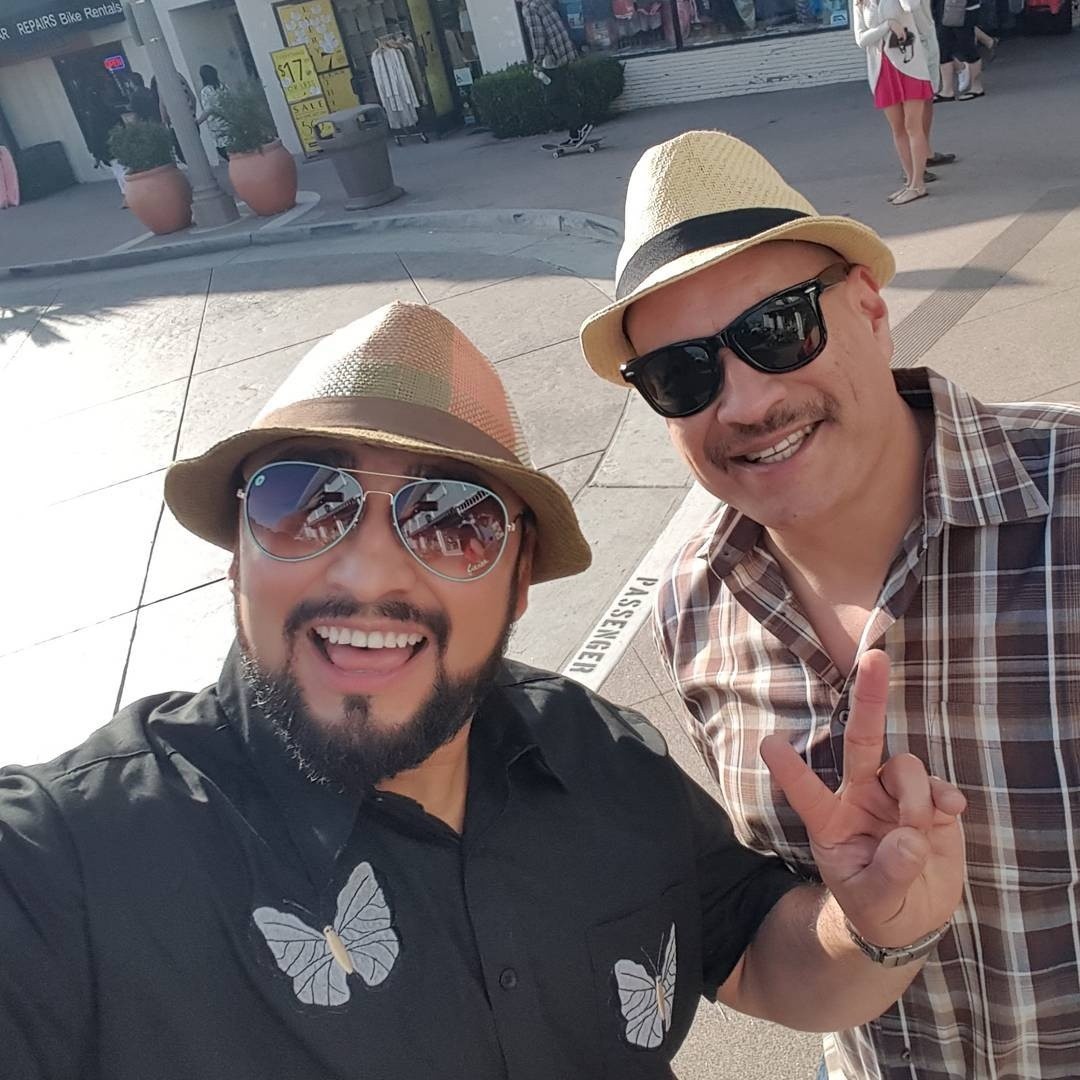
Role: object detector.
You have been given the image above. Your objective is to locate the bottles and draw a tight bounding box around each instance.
[538,72,552,85]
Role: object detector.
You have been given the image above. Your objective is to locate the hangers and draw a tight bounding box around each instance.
[376,30,407,47]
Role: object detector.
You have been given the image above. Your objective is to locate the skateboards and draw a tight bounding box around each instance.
[540,135,607,159]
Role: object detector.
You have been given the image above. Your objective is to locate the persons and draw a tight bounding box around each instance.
[580,129,1080,1080]
[853,0,1000,205]
[89,93,127,208]
[128,70,165,129]
[159,71,196,167]
[196,65,231,162]
[1,300,966,1080]
[522,0,593,148]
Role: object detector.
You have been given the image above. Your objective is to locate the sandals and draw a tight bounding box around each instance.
[888,187,908,200]
[892,188,928,204]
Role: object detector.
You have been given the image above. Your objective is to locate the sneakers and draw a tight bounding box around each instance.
[576,123,593,148]
[559,137,581,146]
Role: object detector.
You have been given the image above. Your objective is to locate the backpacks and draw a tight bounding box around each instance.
[81,107,112,168]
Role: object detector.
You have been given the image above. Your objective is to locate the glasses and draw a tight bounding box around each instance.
[235,460,518,582]
[620,264,848,419]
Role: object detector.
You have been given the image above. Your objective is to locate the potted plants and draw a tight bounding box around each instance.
[105,116,193,236]
[204,73,298,218]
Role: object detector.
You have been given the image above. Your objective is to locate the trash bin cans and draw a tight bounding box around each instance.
[312,105,404,210]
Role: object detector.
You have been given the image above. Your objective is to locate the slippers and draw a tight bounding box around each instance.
[959,91,985,101]
[933,94,955,103]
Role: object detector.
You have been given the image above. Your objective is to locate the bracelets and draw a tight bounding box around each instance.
[844,915,951,968]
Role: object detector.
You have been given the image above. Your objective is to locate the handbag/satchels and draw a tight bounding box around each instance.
[942,0,966,27]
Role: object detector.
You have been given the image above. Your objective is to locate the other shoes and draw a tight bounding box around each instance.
[987,37,999,62]
[903,170,937,185]
[958,62,970,92]
[926,152,956,167]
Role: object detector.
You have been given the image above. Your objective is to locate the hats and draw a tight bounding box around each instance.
[582,130,897,388]
[165,302,592,582]
